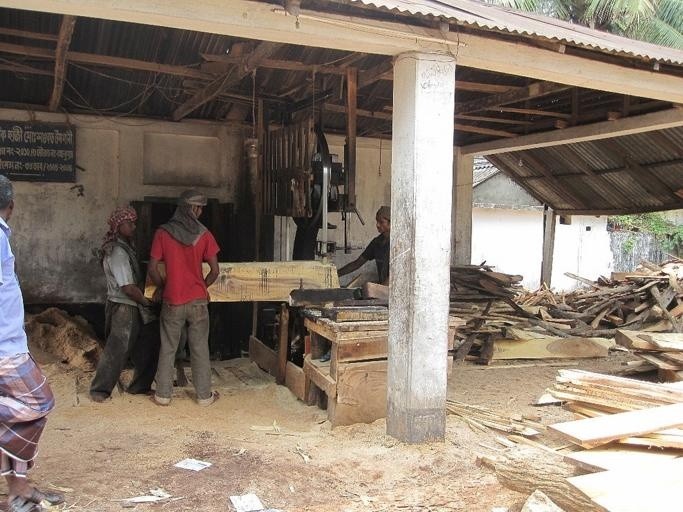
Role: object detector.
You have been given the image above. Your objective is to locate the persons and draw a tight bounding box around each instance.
[0,175,63,512]
[88,206,160,404]
[149,191,221,406]
[337,204,391,287]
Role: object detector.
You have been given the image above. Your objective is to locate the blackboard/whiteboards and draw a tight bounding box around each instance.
[0,119,77,183]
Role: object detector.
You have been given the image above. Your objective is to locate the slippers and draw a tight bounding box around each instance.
[0,494,41,512]
[27,486,65,506]
